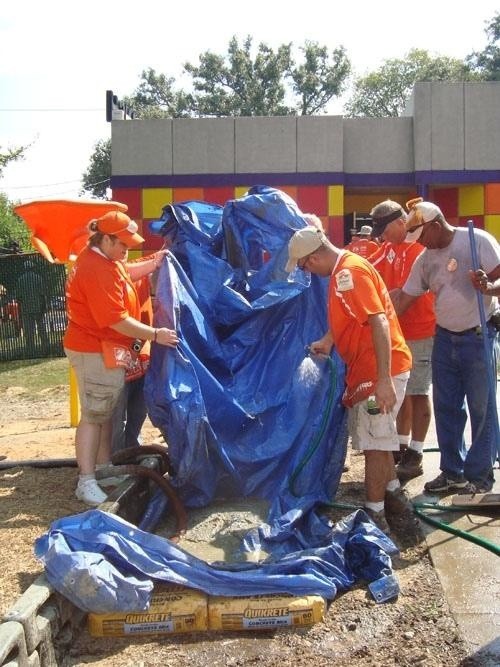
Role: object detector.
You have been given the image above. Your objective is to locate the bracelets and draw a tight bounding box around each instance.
[153,259,158,272]
[154,327,159,343]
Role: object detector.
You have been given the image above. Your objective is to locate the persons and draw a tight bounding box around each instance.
[365,199,457,483]
[15,260,51,359]
[283,225,413,534]
[383,201,500,496]
[63,209,184,513]
[355,224,381,244]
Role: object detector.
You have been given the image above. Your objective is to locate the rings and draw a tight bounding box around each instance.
[480,280,481,284]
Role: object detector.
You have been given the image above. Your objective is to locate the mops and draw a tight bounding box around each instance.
[435,220,500,509]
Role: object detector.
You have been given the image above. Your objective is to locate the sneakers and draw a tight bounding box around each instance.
[365,448,492,534]
[75,479,108,506]
[96,465,130,486]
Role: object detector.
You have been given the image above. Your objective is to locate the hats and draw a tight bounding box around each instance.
[357,202,441,243]
[98,210,145,247]
[284,227,327,272]
